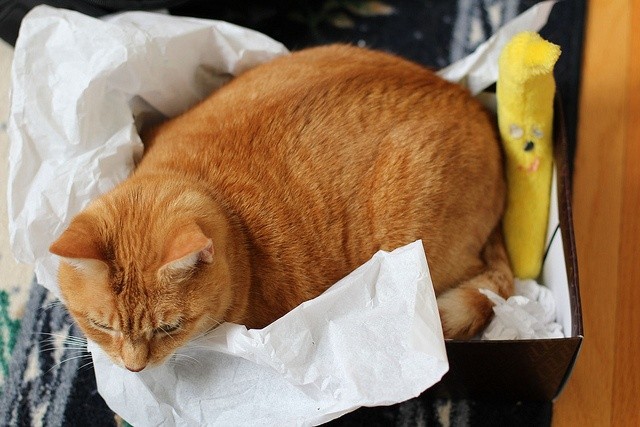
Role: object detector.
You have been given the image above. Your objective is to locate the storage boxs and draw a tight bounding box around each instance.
[407,85,587,404]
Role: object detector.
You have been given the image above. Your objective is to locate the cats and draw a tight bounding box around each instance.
[30,40,515,372]
